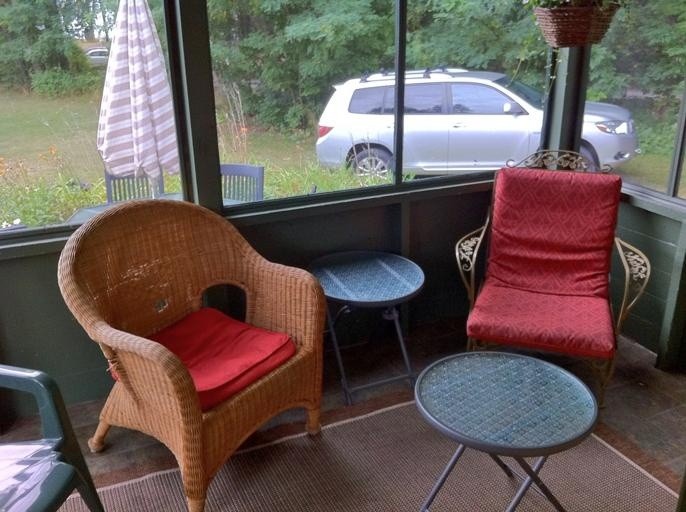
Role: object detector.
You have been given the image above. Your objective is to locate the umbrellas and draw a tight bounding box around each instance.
[96,0,182,198]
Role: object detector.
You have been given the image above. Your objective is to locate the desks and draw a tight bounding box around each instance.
[413,350,601,512]
[309,250,426,409]
[65,193,243,225]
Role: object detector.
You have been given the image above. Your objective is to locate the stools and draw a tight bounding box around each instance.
[0,360,106,512]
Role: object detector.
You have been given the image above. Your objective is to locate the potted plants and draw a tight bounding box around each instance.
[504,0,634,108]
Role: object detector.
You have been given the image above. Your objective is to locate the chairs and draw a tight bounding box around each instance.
[55,196,327,511]
[455,146,652,411]
[220,163,265,201]
[104,167,164,204]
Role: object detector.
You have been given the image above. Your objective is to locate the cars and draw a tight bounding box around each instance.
[83,47,109,69]
[314,67,639,189]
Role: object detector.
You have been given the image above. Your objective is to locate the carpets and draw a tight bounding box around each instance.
[52,397,682,511]
